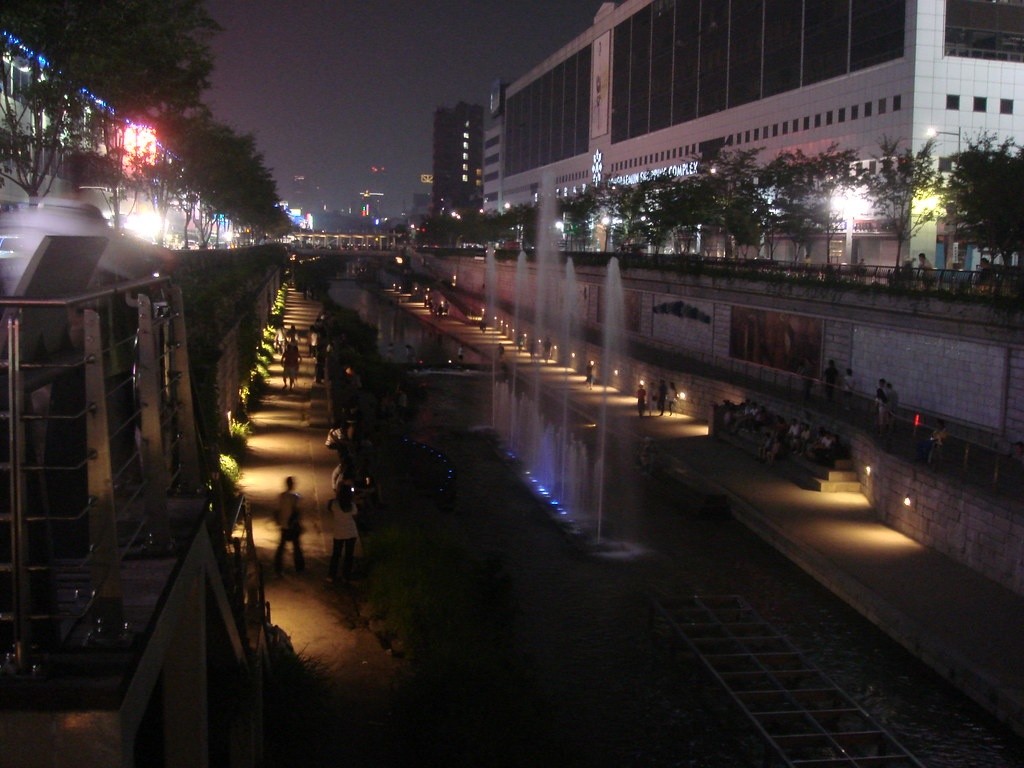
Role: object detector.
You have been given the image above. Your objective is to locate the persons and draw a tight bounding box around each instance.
[804,254,991,291]
[736,345,816,394]
[259,236,267,245]
[1006,441,1024,500]
[872,378,898,436]
[722,398,850,474]
[268,475,305,575]
[932,419,948,451]
[843,369,855,411]
[273,241,676,583]
[824,359,839,400]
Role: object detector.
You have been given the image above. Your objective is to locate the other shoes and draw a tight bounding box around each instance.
[799,452,803,456]
[793,450,799,454]
[754,458,759,461]
[759,460,765,464]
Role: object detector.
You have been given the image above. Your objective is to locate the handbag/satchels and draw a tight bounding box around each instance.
[288,506,302,539]
